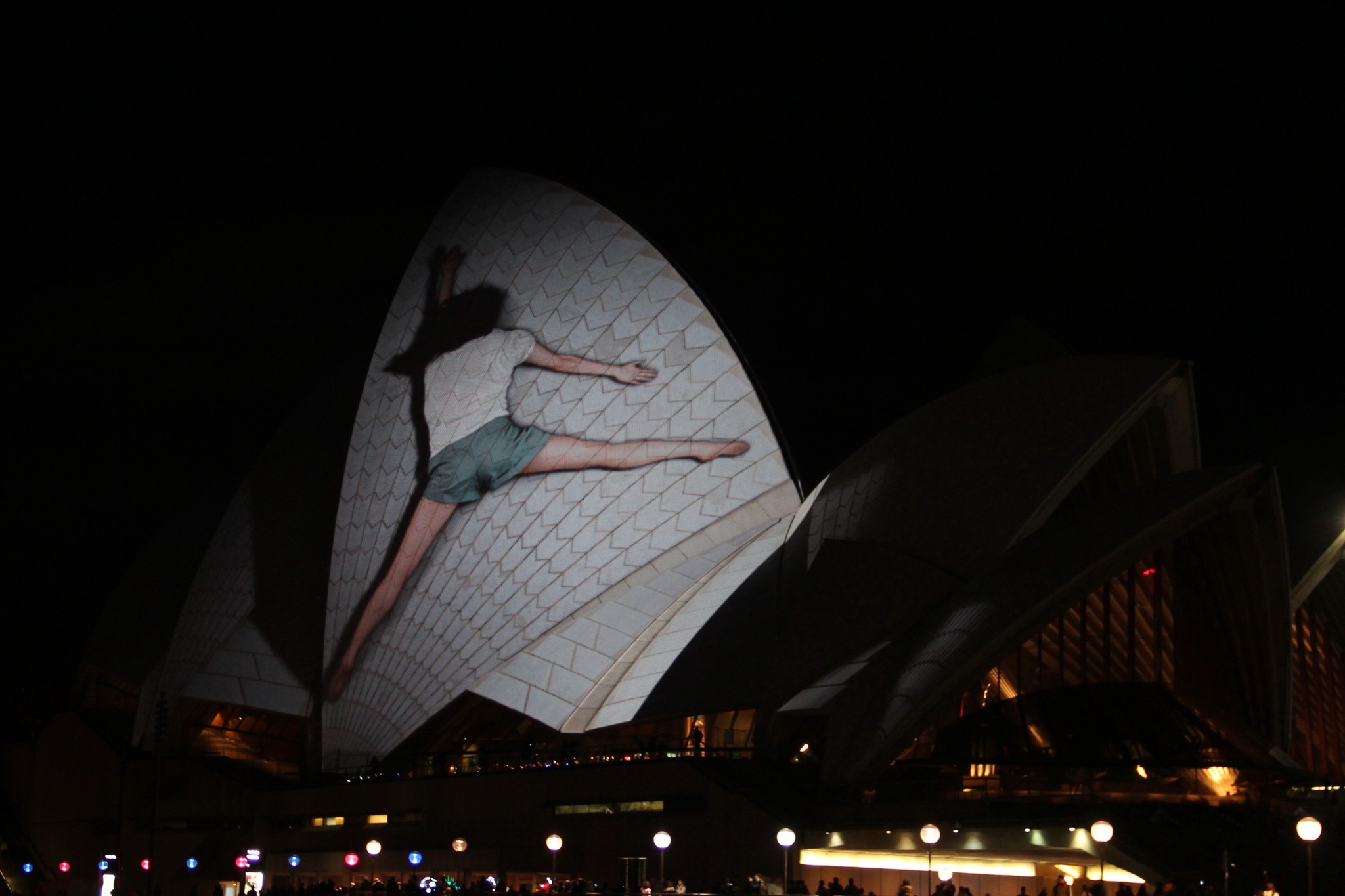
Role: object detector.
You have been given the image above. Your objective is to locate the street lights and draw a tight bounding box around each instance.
[653,831,672,891]
[1090,820,1113,882]
[452,837,468,883]
[919,824,944,896]
[544,834,563,880]
[1295,815,1324,896]
[366,840,382,882]
[235,855,249,896]
[776,828,796,894]
[343,853,360,891]
[288,854,300,887]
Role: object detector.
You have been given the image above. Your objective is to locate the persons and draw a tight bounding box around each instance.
[1260,882,1279,896]
[719,874,784,895]
[1081,882,1171,896]
[1054,875,1071,896]
[897,879,912,896]
[247,884,297,896]
[297,878,347,896]
[213,884,223,896]
[931,879,972,896]
[640,876,686,896]
[359,879,385,892]
[787,880,810,894]
[548,878,595,896]
[1017,886,1048,896]
[815,877,877,896]
[693,727,703,749]
[469,876,494,892]
[386,875,448,896]
